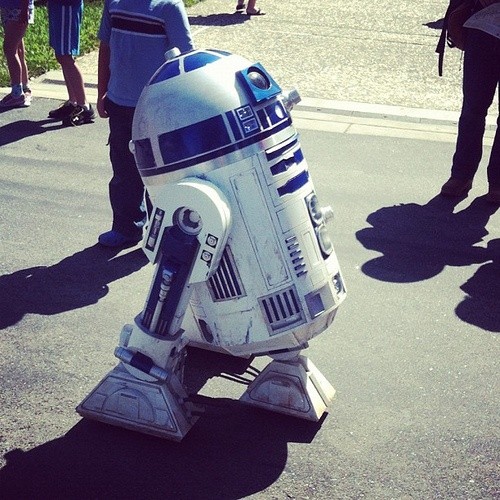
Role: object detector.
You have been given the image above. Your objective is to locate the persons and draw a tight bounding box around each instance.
[441,0,500,203]
[47,0,96,126]
[236,0,264,15]
[0,0,35,107]
[96,0,194,248]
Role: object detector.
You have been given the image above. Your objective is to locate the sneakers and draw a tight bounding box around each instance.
[20,90,33,107]
[0,92,27,108]
[63,104,97,126]
[48,102,80,118]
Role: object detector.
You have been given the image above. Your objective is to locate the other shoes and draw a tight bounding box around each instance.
[487,180,500,204]
[441,179,472,197]
[100,229,143,246]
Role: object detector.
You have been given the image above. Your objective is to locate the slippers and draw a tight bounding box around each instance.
[237,4,247,9]
[248,8,266,15]
